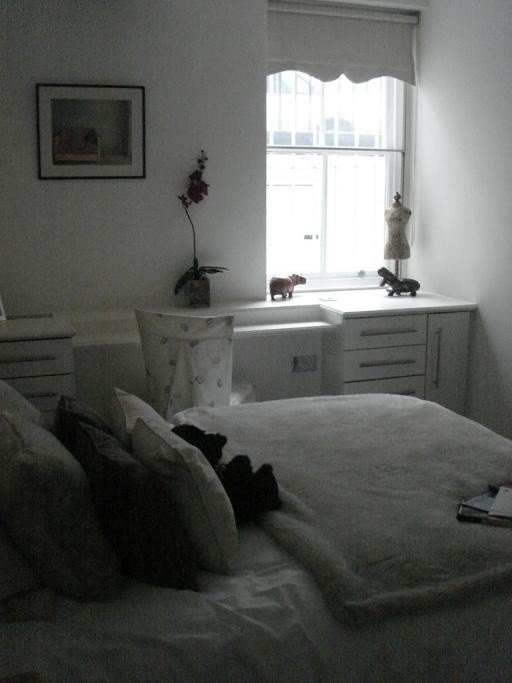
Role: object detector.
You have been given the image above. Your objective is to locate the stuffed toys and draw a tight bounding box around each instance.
[171,425,279,526]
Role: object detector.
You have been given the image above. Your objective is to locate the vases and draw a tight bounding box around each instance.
[184,271,210,309]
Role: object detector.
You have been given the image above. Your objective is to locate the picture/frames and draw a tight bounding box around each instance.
[34,81,147,180]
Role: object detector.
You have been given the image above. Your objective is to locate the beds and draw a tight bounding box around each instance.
[0,374,511,683]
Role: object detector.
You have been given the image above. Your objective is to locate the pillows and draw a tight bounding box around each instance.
[2,381,240,603]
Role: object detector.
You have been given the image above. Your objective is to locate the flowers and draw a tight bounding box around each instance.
[169,147,234,296]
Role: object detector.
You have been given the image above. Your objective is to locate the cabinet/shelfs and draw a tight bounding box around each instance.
[1,309,80,429]
[314,285,478,417]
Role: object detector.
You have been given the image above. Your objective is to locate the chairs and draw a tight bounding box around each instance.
[132,307,257,422]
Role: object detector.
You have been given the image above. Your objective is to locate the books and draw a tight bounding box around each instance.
[456,486,512,527]
